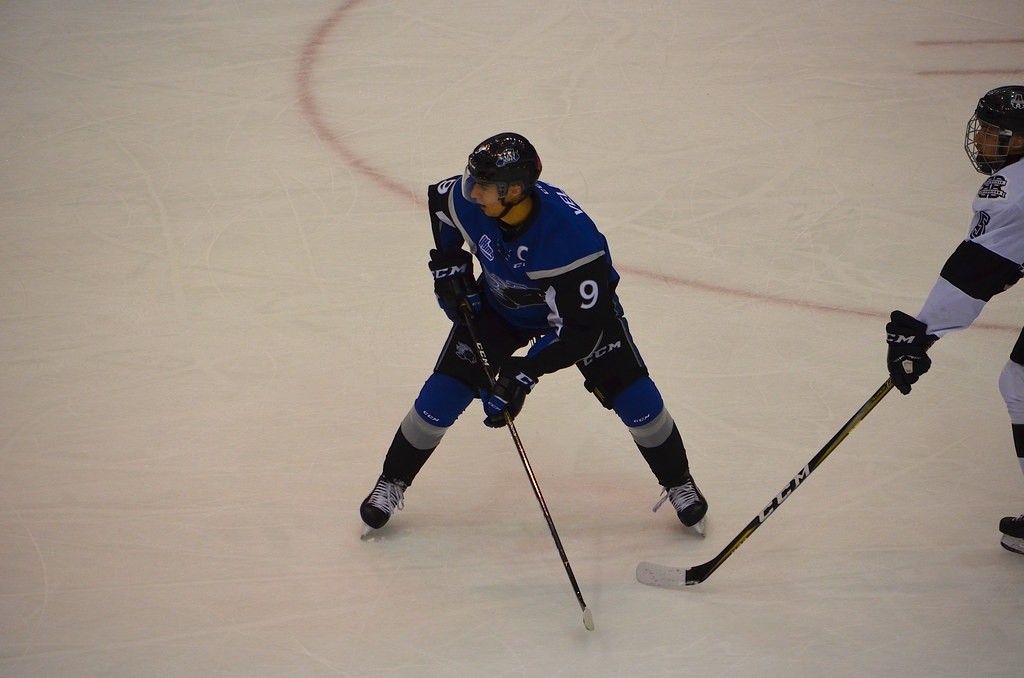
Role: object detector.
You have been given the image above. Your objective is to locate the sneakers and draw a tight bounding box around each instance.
[652,475,708,538]
[999,514,1024,553]
[360,469,407,539]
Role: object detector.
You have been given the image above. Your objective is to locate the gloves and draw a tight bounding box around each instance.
[886,311,939,395]
[428,250,483,332]
[484,359,532,427]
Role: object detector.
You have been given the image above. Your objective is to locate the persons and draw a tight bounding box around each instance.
[888,86,1024,558]
[361,132,708,538]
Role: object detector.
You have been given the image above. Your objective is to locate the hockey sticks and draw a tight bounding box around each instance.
[458,301,594,632]
[634,328,948,590]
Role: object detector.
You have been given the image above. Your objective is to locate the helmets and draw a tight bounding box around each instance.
[963,86,1024,175]
[462,131,542,203]
